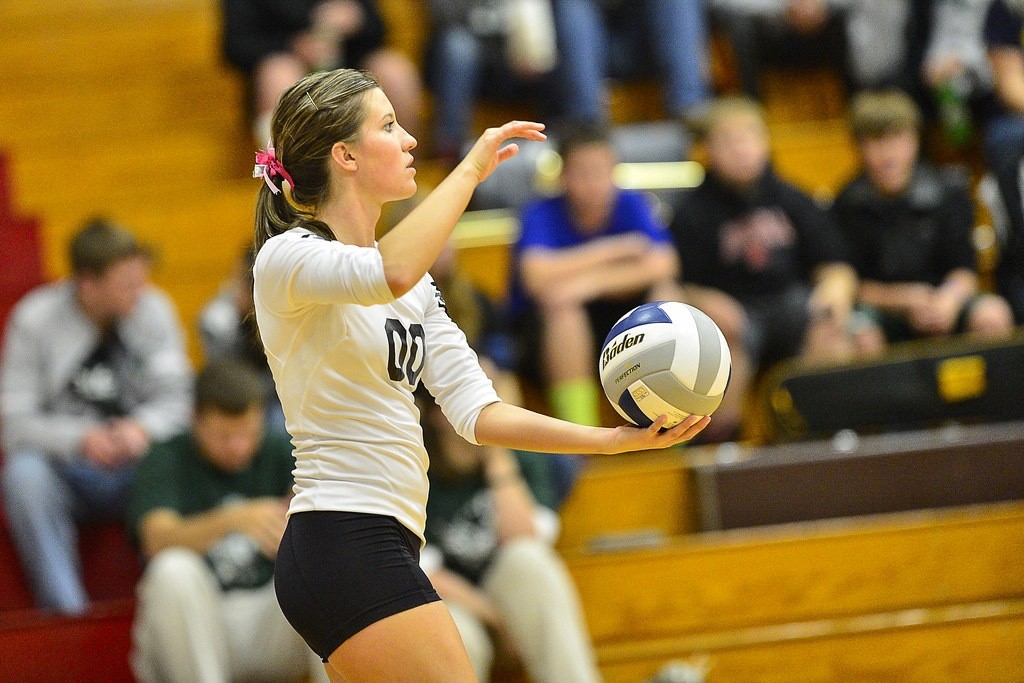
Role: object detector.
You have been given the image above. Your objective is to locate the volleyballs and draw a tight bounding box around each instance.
[598,302,733,428]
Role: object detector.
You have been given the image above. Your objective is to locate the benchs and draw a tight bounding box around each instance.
[0,0,1024,683]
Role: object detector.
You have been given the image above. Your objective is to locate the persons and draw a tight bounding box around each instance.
[251,64,711,683]
[1,2,1024,682]
